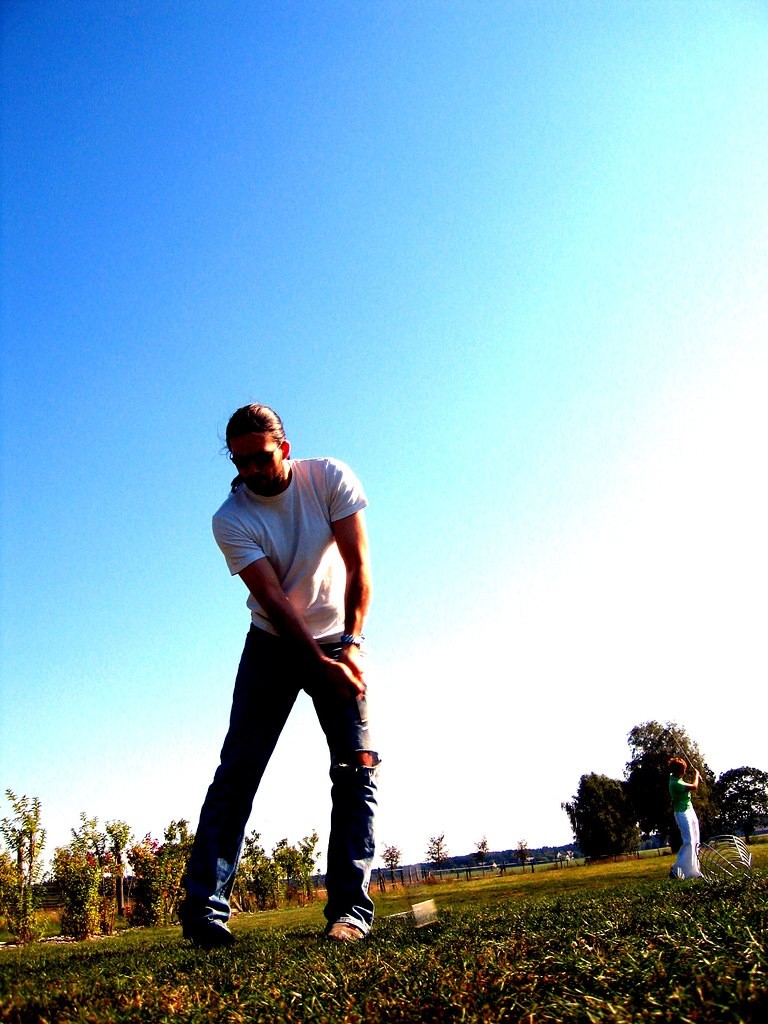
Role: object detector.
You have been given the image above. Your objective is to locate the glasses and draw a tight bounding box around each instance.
[230,445,281,468]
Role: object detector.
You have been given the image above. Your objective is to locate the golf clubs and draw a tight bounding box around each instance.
[669,728,695,770]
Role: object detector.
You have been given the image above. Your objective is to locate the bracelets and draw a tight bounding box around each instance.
[340,634,361,649]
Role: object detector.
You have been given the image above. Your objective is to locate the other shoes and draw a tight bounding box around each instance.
[667,868,677,879]
[324,920,364,942]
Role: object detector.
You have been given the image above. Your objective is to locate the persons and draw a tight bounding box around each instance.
[176,404,382,946]
[668,758,705,880]
